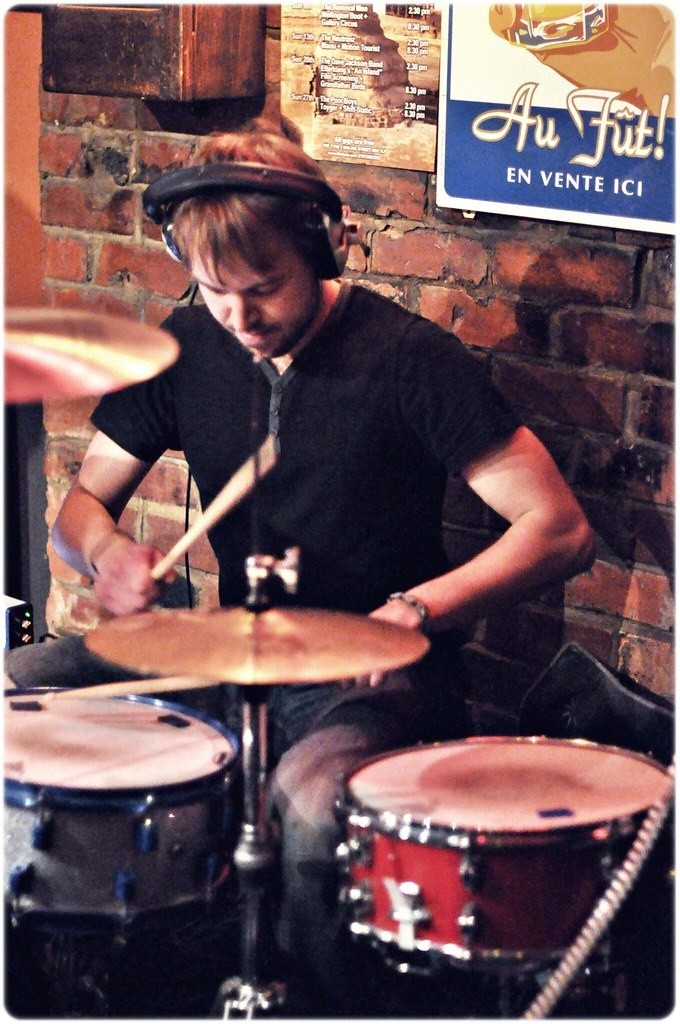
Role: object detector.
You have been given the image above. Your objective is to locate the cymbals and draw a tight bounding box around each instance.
[3,303,184,408]
[81,602,434,688]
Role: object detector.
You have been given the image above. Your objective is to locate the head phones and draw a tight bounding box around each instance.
[141,161,369,282]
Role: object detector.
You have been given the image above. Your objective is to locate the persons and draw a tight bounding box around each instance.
[4,133,594,956]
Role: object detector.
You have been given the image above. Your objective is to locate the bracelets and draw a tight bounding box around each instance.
[90,529,133,572]
[387,592,431,633]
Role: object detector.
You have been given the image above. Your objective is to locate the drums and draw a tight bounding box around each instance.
[326,729,675,988]
[3,679,254,926]
